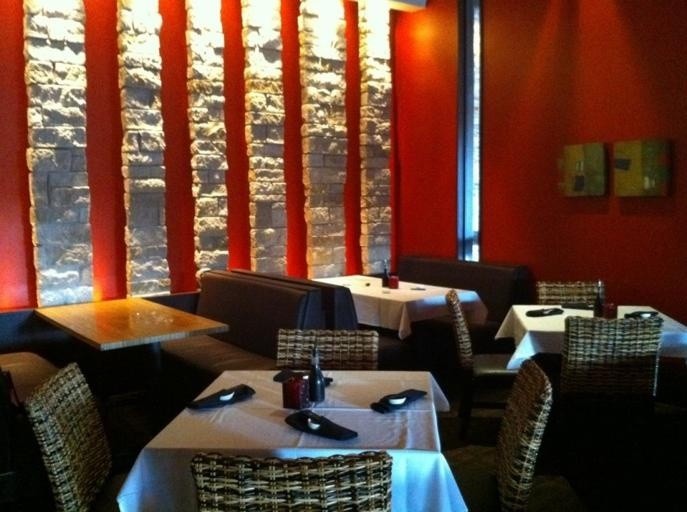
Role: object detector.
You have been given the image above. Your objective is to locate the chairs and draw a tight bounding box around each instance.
[447,291,517,430]
[537,279,605,304]
[25,362,127,512]
[189,451,391,512]
[277,328,380,369]
[496,359,553,512]
[561,316,664,400]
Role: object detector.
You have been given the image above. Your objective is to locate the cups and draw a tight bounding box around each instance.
[388,271,400,289]
[602,302,617,319]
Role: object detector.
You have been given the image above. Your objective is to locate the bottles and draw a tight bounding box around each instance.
[593,279,604,318]
[380,259,389,288]
[307,340,325,400]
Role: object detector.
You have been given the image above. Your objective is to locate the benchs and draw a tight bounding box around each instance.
[396,255,525,352]
[161,272,325,379]
[232,267,416,370]
[2,348,61,406]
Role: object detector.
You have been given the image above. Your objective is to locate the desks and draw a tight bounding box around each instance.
[33,298,230,351]
